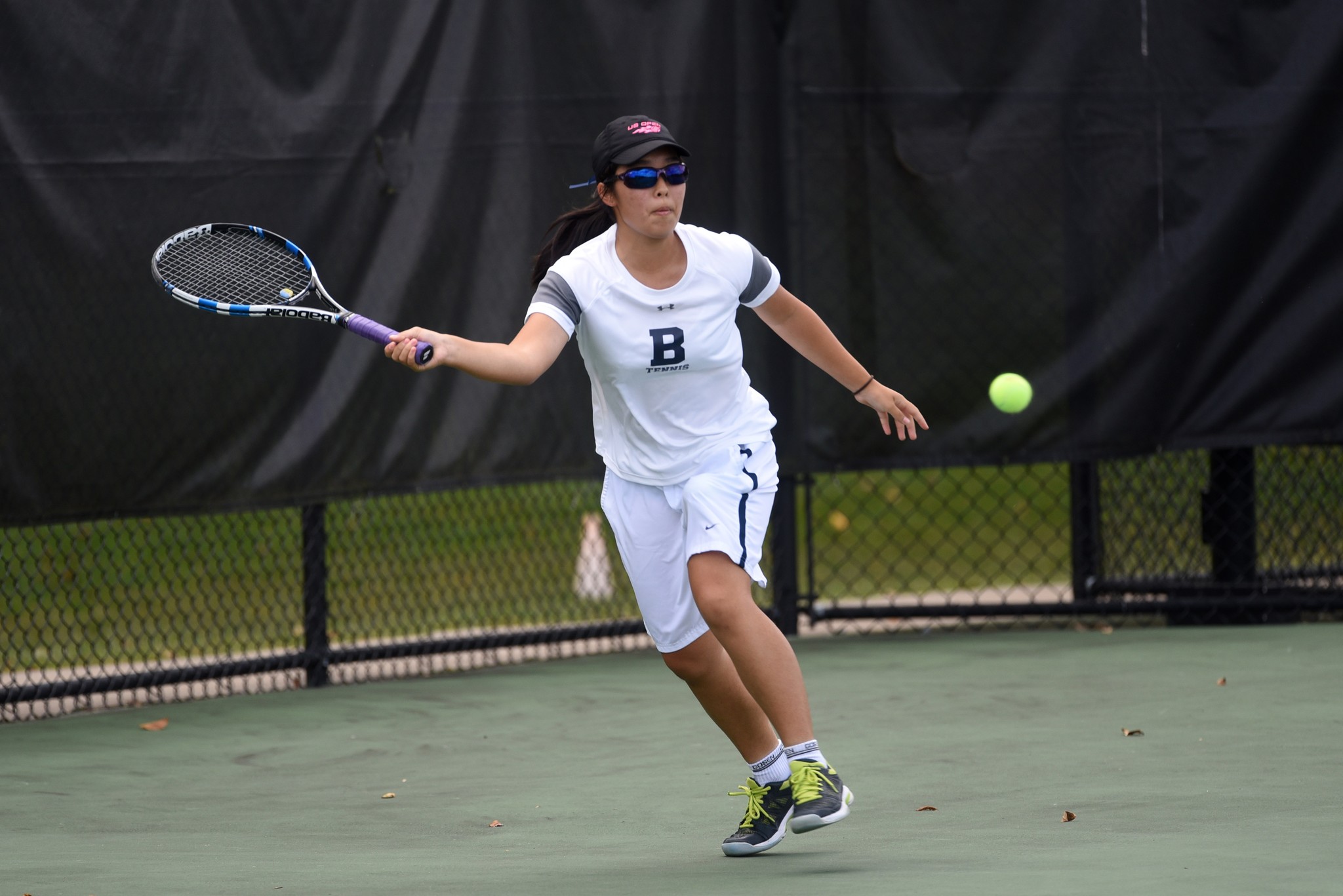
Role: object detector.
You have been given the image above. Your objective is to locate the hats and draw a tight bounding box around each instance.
[589,116,691,186]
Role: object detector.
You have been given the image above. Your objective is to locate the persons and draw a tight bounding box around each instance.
[384,114,929,854]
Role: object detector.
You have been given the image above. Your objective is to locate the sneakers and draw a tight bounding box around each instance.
[788,757,852,833]
[722,774,791,856]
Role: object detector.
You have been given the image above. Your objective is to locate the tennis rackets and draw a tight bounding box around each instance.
[148,221,436,367]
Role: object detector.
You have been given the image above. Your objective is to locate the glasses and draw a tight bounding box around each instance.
[609,163,689,189]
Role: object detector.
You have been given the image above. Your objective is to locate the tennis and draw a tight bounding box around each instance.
[988,372,1034,415]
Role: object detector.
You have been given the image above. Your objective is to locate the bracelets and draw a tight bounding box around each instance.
[853,375,874,395]
